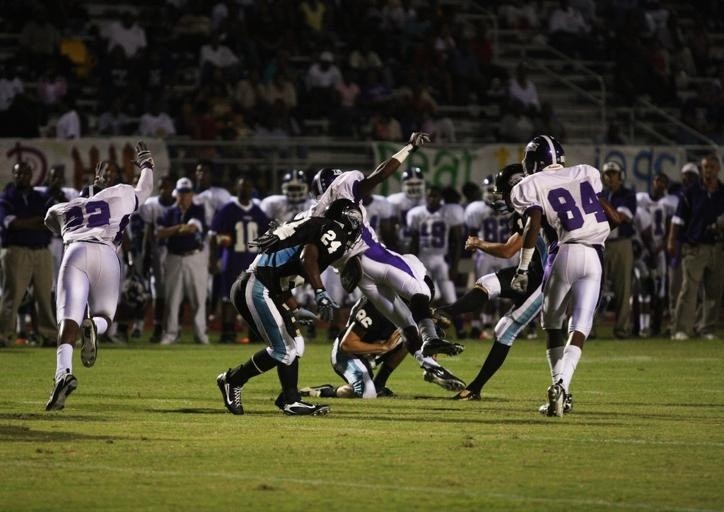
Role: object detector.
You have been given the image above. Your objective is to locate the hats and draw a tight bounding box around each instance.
[603,160,621,174]
[320,51,334,62]
[681,162,700,176]
[175,177,193,192]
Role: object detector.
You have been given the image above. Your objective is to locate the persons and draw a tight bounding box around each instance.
[217,135,610,414]
[45,140,155,412]
[1,0,722,343]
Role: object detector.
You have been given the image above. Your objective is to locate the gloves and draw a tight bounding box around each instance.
[93,159,112,188]
[130,140,155,169]
[409,130,434,149]
[315,286,340,321]
[510,268,529,293]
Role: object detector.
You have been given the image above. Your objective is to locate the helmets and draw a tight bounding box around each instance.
[482,164,523,215]
[282,167,364,251]
[521,134,564,176]
[400,166,426,198]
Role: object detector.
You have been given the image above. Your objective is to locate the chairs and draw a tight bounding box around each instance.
[1,0,724,144]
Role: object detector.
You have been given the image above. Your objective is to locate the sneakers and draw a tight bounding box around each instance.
[159,334,178,345]
[538,383,572,418]
[193,334,210,345]
[703,332,718,341]
[80,317,98,367]
[588,327,597,339]
[1,326,59,351]
[105,324,161,345]
[216,306,482,416]
[613,326,632,339]
[670,329,690,341]
[46,372,77,411]
